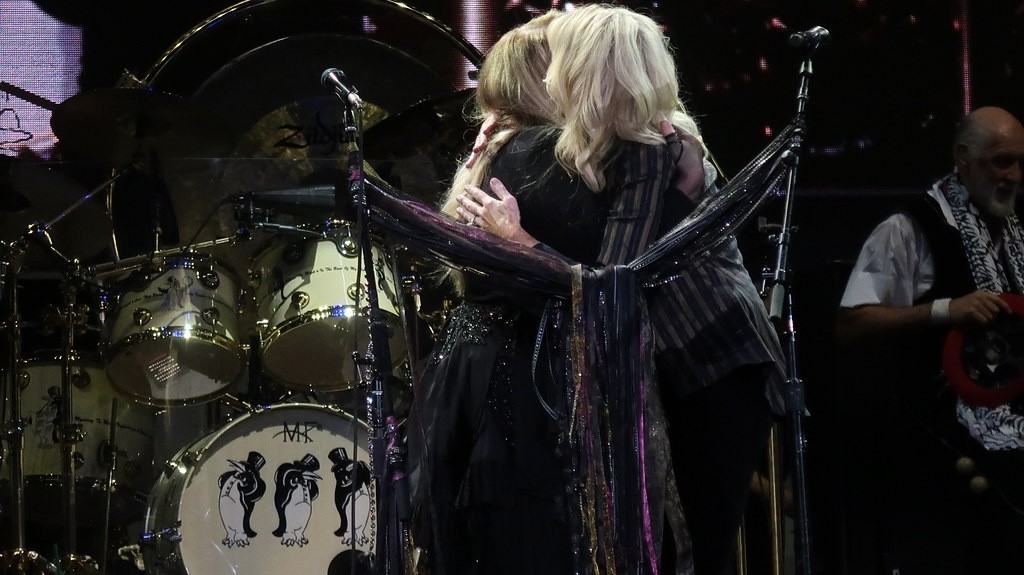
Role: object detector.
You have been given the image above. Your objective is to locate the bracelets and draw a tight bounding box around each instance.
[931,299,951,321]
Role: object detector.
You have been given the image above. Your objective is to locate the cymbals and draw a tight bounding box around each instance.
[50,86,233,179]
[0,153,114,269]
[359,86,477,154]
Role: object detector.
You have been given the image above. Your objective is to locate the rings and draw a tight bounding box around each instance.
[467,214,476,226]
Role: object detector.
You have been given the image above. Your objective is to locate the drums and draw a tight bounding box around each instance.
[98,251,243,410]
[244,220,411,395]
[6,344,146,498]
[141,397,399,575]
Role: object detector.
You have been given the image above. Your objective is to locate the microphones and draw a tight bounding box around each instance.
[784,25,832,49]
[321,66,362,107]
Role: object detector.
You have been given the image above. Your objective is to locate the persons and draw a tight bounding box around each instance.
[407,3,790,575]
[836,106,1024,574]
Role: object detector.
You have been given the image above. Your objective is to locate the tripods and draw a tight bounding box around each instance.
[1,156,132,575]
